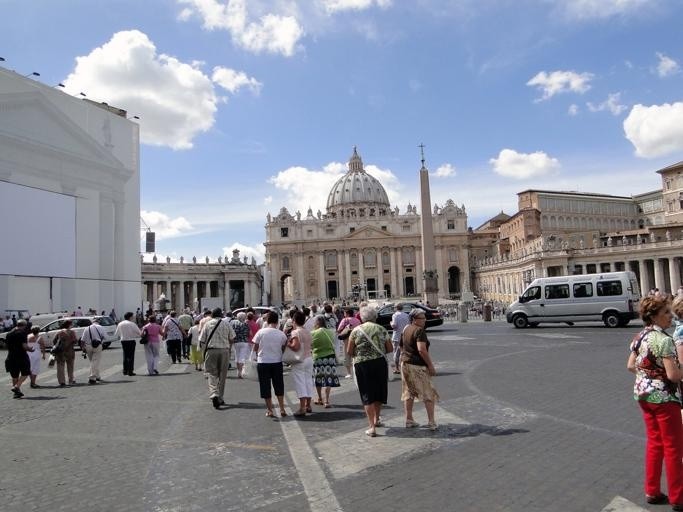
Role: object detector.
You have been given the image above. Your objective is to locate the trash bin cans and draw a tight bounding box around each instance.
[483,304,491,321]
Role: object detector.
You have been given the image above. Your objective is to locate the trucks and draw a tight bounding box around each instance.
[0,312,69,346]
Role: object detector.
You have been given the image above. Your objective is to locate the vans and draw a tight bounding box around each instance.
[229,306,282,317]
[505,271,642,327]
[26,315,120,351]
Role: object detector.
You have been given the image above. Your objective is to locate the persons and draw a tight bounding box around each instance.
[627,293,683,512]
[648,284,683,366]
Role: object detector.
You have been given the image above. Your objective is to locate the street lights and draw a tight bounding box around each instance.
[159,290,167,310]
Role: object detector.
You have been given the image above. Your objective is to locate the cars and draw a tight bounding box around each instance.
[340,304,360,314]
[376,302,445,332]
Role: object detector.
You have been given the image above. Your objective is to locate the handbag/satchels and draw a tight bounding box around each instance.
[338,317,352,340]
[280,330,305,363]
[50,333,65,353]
[387,359,394,382]
[91,339,101,349]
[241,343,259,381]
[139,327,149,344]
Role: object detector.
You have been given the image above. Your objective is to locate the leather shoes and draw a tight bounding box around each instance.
[29,368,159,388]
[345,374,352,379]
[171,353,204,371]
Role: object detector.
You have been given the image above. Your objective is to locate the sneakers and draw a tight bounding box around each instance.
[212,396,220,408]
[646,494,669,505]
[672,505,683,512]
[365,430,376,437]
[265,400,331,417]
[375,416,381,425]
[13,394,20,398]
[406,421,419,428]
[11,386,24,396]
[429,423,439,431]
